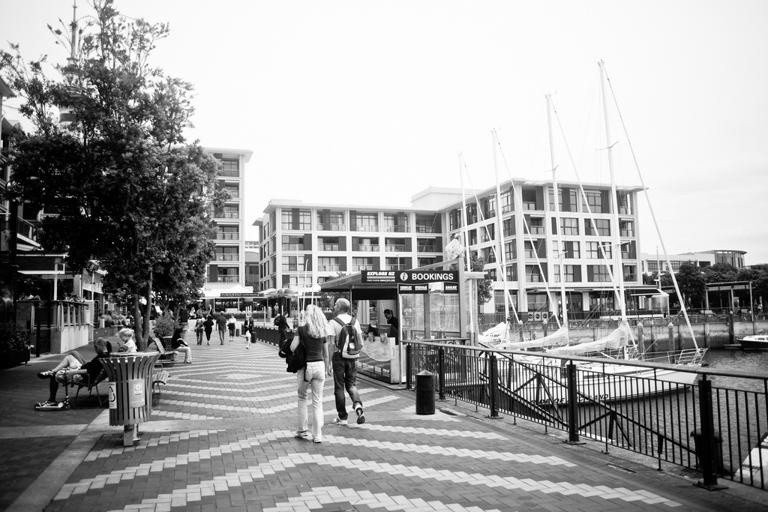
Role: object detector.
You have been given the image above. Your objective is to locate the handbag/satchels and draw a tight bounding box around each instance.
[278,339,308,373]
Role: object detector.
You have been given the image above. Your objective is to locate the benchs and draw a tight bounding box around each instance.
[151,363,169,401]
[58,365,107,406]
[149,332,178,366]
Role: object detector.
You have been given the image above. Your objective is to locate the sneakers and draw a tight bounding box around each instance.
[354,403,366,424]
[311,432,323,444]
[294,431,308,439]
[41,400,58,406]
[332,415,348,426]
[37,369,55,380]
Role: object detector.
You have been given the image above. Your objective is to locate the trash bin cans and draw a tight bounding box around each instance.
[98,351,161,446]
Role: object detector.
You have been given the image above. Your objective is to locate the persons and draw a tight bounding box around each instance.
[187,301,291,352]
[384,309,404,344]
[115,326,138,353]
[170,326,192,364]
[289,304,330,443]
[34,336,114,412]
[97,309,137,328]
[326,297,367,426]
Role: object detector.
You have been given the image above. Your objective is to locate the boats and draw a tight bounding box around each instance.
[735,334,768,350]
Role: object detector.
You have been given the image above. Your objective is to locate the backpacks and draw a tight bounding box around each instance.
[333,313,364,360]
